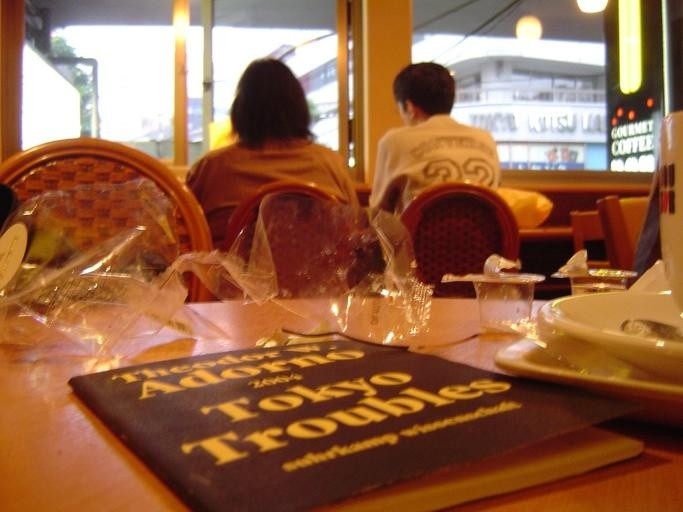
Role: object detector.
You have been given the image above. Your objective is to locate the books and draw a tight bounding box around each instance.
[66,338,645,511]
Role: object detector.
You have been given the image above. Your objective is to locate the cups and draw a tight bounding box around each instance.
[568,264,638,297]
[473,272,547,337]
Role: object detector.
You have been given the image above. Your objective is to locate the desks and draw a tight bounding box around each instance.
[0,296,681,512]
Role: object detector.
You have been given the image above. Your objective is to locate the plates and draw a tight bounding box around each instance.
[537,290,683,384]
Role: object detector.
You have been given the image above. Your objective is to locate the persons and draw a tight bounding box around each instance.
[369,63,502,224]
[167,58,363,252]
